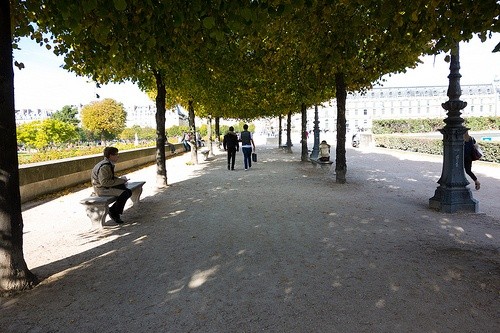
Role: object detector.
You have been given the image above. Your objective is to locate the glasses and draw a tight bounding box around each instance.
[111,153,119,157]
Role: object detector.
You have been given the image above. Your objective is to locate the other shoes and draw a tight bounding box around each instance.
[108,208,124,224]
[475,181,480,190]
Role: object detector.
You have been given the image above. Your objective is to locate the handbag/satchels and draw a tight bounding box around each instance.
[471,137,482,161]
[111,176,127,190]
[252,153,257,162]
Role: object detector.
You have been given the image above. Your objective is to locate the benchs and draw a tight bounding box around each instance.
[309,156,333,169]
[280,143,293,153]
[201,150,210,161]
[80,181,147,228]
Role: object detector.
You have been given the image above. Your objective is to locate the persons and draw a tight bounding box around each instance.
[165,133,179,154]
[319,140,331,161]
[223,126,239,169]
[181,129,193,152]
[305,129,309,140]
[196,134,203,147]
[238,124,256,170]
[91,147,132,224]
[461,126,482,191]
